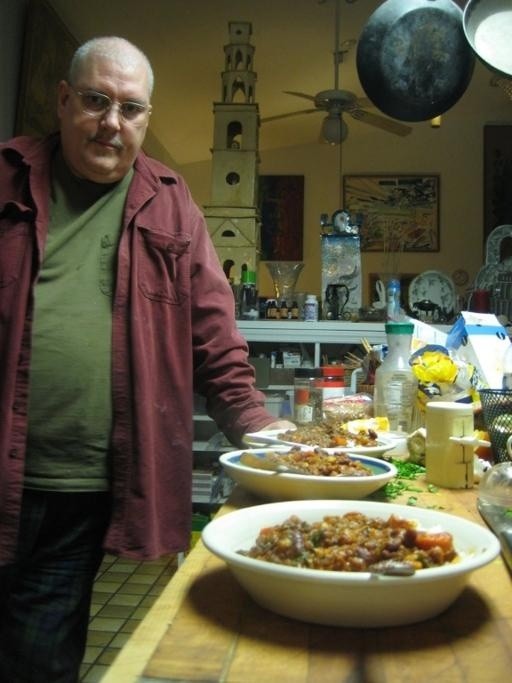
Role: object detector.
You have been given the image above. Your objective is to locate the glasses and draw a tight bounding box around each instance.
[71,85,152,128]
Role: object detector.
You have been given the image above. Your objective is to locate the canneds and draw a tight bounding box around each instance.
[293,366,347,429]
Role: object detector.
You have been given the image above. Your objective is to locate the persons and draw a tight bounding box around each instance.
[0,34,307,683]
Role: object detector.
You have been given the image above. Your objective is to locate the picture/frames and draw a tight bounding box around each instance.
[260,175,305,262]
[483,124,512,265]
[14,0,81,137]
[342,174,441,253]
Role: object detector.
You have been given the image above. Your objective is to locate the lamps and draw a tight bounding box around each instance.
[317,112,353,147]
[431,115,441,129]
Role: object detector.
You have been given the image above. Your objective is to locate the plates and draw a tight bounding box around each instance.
[407,269,457,320]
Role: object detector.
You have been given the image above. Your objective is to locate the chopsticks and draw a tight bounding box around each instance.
[343,338,373,370]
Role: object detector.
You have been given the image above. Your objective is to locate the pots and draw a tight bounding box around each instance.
[356,0,512,123]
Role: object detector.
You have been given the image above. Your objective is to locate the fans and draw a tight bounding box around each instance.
[260,0,413,137]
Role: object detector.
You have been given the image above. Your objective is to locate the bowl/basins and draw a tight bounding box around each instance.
[200,499,501,629]
[217,447,399,498]
[242,427,396,458]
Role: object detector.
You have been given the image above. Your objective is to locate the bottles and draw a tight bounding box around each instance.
[294,366,346,426]
[239,270,320,321]
[386,278,401,321]
[373,321,418,433]
[502,336,512,391]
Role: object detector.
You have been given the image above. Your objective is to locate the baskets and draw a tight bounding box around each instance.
[479,389,512,463]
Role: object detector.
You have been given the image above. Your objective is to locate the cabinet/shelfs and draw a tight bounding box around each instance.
[192,319,512,503]
